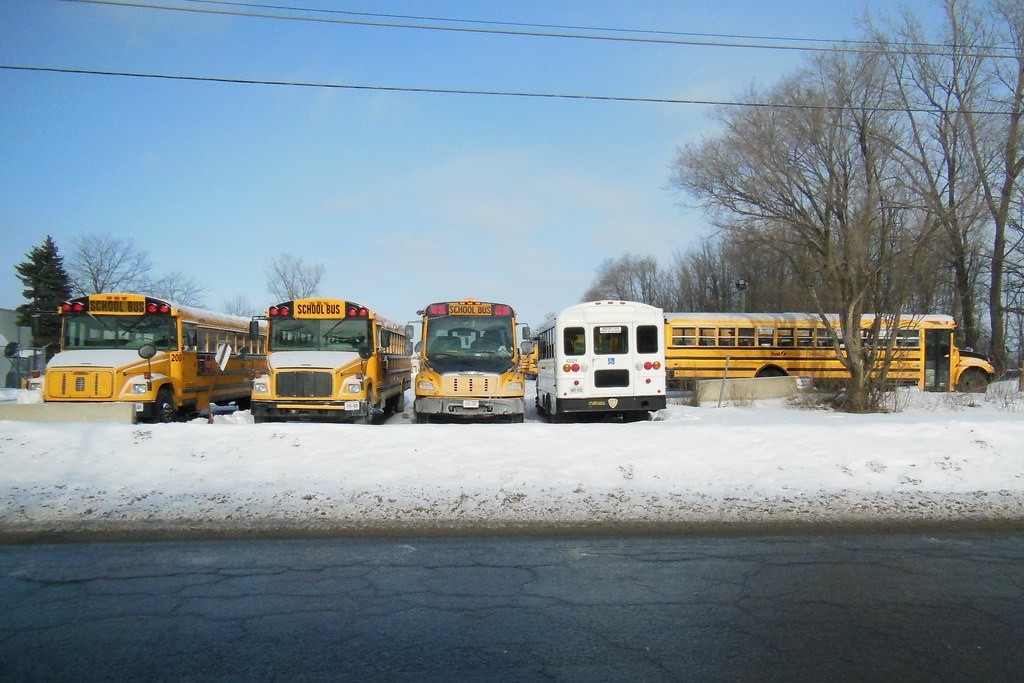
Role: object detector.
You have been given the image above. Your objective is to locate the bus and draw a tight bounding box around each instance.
[531,300,667,424]
[664,314,996,393]
[405,299,531,423]
[249,299,412,424]
[44,293,267,425]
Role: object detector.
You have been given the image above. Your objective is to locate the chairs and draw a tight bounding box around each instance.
[431,336,461,354]
[470,337,500,351]
[676,341,831,346]
